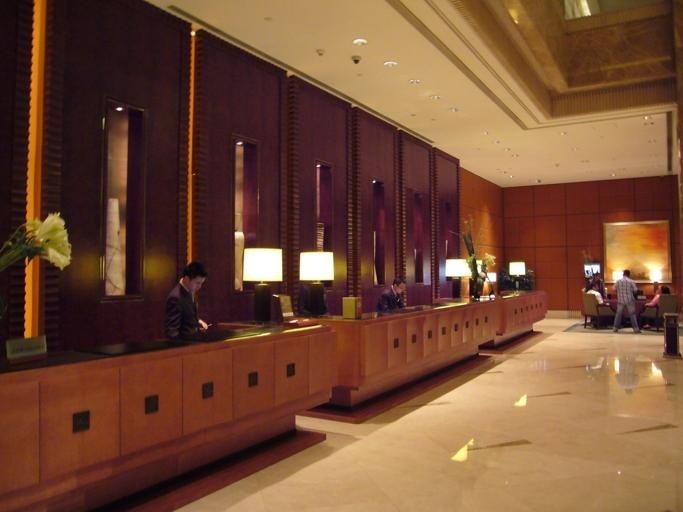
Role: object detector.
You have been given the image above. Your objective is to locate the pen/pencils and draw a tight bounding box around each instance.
[201,323,214,330]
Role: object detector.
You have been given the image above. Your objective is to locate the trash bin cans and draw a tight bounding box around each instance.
[663,312,679,359]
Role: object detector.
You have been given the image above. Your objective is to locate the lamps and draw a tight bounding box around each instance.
[244,248,282,322]
[300,252,335,313]
[446,259,471,297]
[509,261,526,290]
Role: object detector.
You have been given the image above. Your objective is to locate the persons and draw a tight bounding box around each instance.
[639,284,668,329]
[584,272,606,297]
[588,282,609,330]
[155,260,209,342]
[377,277,406,313]
[609,269,641,334]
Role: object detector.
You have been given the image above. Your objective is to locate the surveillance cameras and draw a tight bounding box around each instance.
[352,56,360,64]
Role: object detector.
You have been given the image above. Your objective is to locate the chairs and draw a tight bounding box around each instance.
[581,290,677,331]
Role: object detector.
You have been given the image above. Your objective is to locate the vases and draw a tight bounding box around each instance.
[472,276,484,301]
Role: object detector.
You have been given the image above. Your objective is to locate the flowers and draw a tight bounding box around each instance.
[468,252,496,299]
[0,212,72,271]
